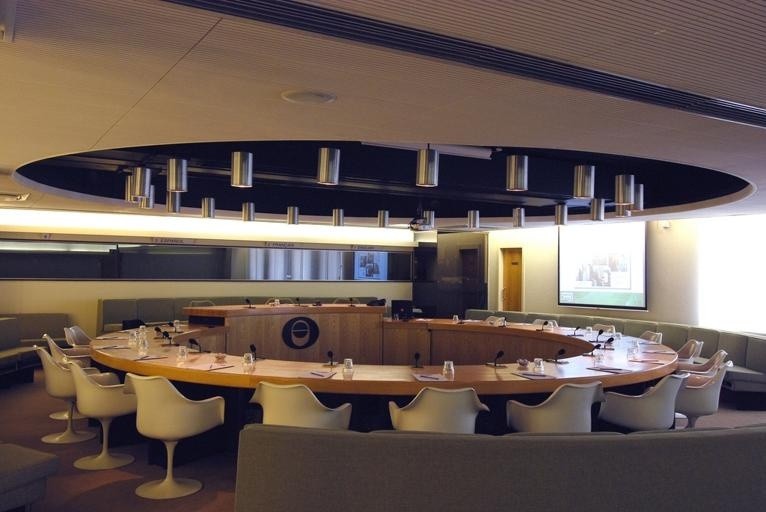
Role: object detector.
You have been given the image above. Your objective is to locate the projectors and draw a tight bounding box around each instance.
[409,217,432,230]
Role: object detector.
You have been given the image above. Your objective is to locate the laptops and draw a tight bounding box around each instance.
[392,300,413,319]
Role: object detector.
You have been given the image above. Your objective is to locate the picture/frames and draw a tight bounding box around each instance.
[558,221,647,311]
[353,251,388,280]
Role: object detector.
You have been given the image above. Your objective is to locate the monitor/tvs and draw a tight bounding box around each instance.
[413,246,480,284]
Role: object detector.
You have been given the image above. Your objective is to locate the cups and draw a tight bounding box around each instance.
[178,345,187,360]
[442,360,455,375]
[173,320,180,330]
[344,359,354,373]
[586,327,640,368]
[243,353,253,366]
[547,322,553,328]
[453,314,459,321]
[532,358,544,373]
[128,325,148,355]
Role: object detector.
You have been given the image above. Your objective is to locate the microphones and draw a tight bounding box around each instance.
[414,353,420,368]
[574,326,580,332]
[604,338,614,347]
[555,349,565,362]
[155,293,353,366]
[542,321,548,327]
[592,344,601,352]
[597,329,603,337]
[494,350,504,365]
